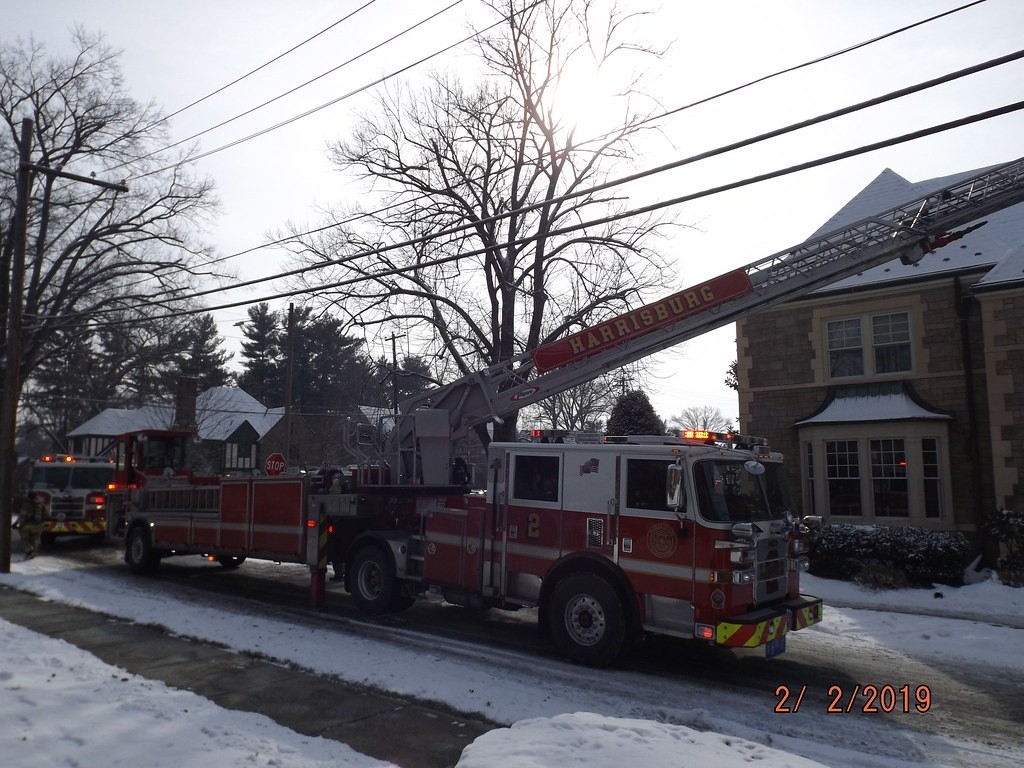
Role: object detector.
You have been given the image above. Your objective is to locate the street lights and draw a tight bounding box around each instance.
[233,320,294,467]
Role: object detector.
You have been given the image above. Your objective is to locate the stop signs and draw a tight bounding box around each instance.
[264,453,286,475]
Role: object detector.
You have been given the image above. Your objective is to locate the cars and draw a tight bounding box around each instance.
[306,465,359,491]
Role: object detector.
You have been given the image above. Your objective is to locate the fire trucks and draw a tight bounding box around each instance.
[21,453,127,545]
[104,157,1024,664]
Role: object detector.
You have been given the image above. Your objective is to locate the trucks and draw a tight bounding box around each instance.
[96,429,221,486]
